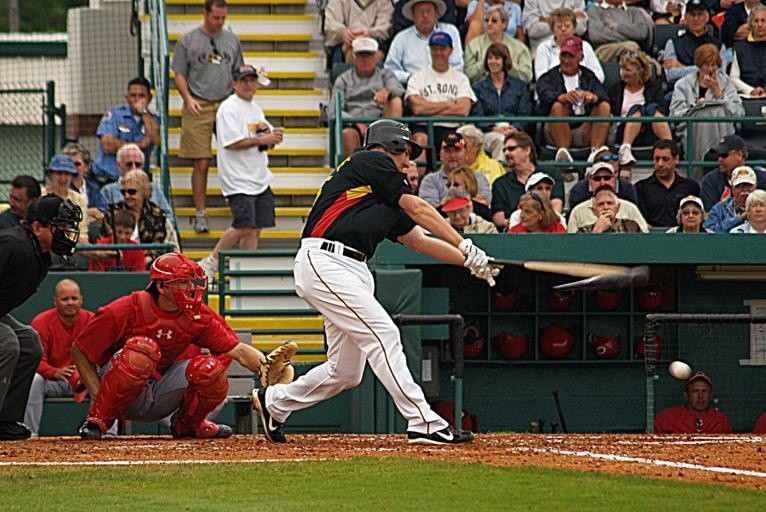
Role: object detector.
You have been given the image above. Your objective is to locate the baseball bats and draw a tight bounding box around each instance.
[465,256,630,281]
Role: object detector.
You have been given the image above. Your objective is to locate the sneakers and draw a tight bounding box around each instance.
[1,420,31,439]
[618,146,637,166]
[555,147,575,182]
[252,387,287,442]
[170,422,232,438]
[196,260,217,291]
[407,425,474,446]
[585,145,609,170]
[193,210,210,233]
[78,421,102,440]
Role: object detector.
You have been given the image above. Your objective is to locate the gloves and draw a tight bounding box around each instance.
[458,237,504,287]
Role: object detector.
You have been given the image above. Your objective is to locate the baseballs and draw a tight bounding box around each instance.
[668,361,693,381]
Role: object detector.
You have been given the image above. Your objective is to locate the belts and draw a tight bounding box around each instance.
[320,242,366,262]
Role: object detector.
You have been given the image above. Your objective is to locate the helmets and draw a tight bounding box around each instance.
[464,326,485,358]
[550,290,571,310]
[69,368,89,403]
[363,117,423,160]
[638,289,663,311]
[590,329,621,359]
[146,251,208,322]
[27,192,83,266]
[492,287,518,310]
[594,287,623,309]
[494,331,529,359]
[638,334,662,358]
[541,321,575,357]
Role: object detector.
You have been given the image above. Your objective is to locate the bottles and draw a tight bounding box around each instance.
[572,87,586,115]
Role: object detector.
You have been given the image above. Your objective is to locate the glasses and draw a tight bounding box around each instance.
[210,39,218,55]
[503,146,519,152]
[624,50,640,59]
[592,176,612,181]
[683,208,699,215]
[120,189,137,195]
[126,160,142,168]
[595,154,619,161]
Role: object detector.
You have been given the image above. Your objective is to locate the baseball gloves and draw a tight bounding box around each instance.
[259,341,298,388]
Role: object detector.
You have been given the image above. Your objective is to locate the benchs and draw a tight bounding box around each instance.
[40,330,261,435]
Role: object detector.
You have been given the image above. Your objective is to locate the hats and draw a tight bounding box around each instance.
[524,171,555,191]
[401,0,448,22]
[730,165,756,187]
[352,37,379,53]
[441,132,467,150]
[233,64,258,80]
[46,154,79,179]
[589,162,614,176]
[429,32,452,47]
[441,197,470,213]
[680,194,704,210]
[686,0,708,12]
[560,36,584,55]
[689,370,713,386]
[710,135,744,155]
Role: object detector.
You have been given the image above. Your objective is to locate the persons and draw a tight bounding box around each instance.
[0,194,82,440]
[252,118,504,449]
[169,2,284,283]
[1,77,180,272]
[17,278,118,439]
[322,1,765,233]
[69,251,269,440]
